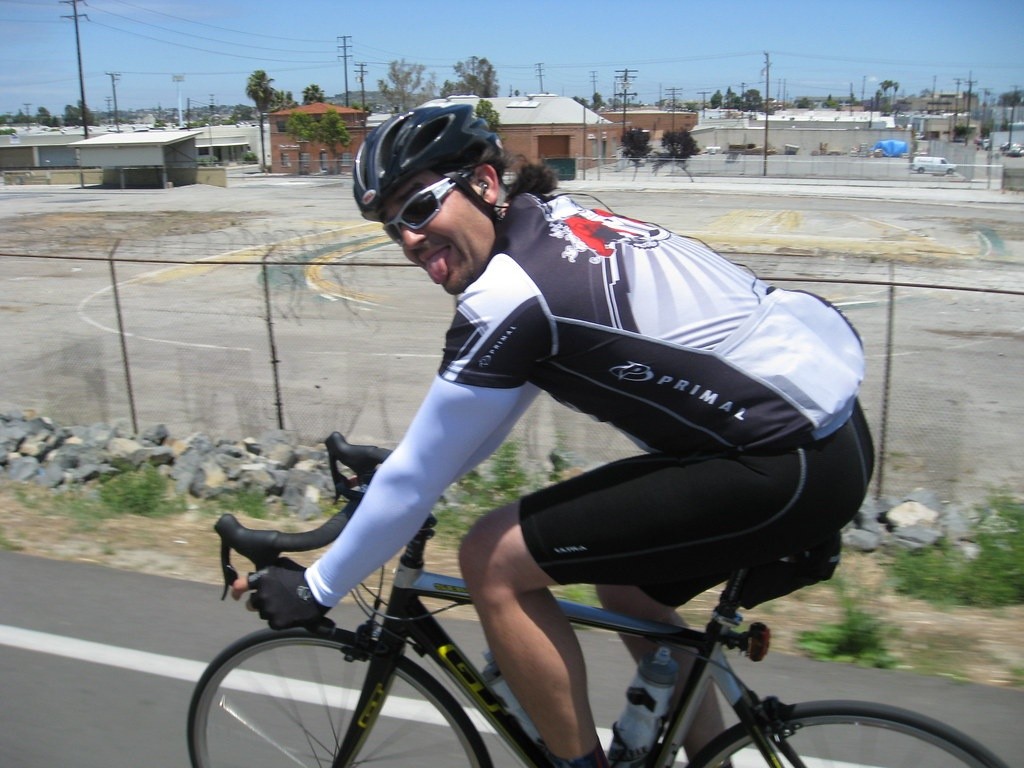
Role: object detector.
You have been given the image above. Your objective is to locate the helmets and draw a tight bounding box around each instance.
[351,94,501,221]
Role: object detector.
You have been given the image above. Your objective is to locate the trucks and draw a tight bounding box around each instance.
[983,131,1024,151]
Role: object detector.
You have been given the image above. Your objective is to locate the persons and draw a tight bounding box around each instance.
[231,94,875,768]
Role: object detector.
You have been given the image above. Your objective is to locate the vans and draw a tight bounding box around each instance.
[912,157,956,174]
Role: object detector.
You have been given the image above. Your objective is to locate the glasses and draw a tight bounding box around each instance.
[382,166,478,248]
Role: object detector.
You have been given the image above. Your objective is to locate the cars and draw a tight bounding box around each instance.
[915,133,924,139]
[3,171,51,186]
[1000,142,1024,157]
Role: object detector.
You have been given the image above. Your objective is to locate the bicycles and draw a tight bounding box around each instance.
[187,431,1013,768]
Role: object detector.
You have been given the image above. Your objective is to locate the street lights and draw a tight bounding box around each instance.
[597,106,605,180]
[209,114,222,165]
[583,104,589,180]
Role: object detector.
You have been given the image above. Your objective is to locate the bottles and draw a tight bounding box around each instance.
[482,650,543,744]
[607,646,685,768]
